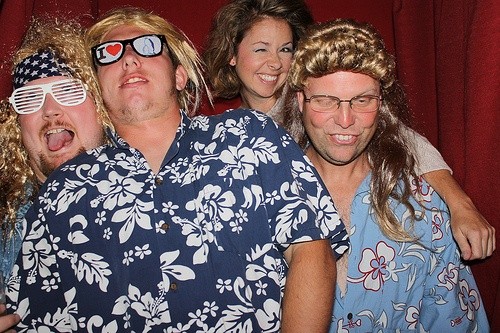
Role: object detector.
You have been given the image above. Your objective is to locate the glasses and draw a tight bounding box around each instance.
[91,34,175,72]
[9,78,90,114]
[304,95,383,113]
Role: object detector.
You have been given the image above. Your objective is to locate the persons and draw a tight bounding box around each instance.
[0,6,352,333]
[203,0,495,260]
[0,9,117,333]
[272,18,491,333]
